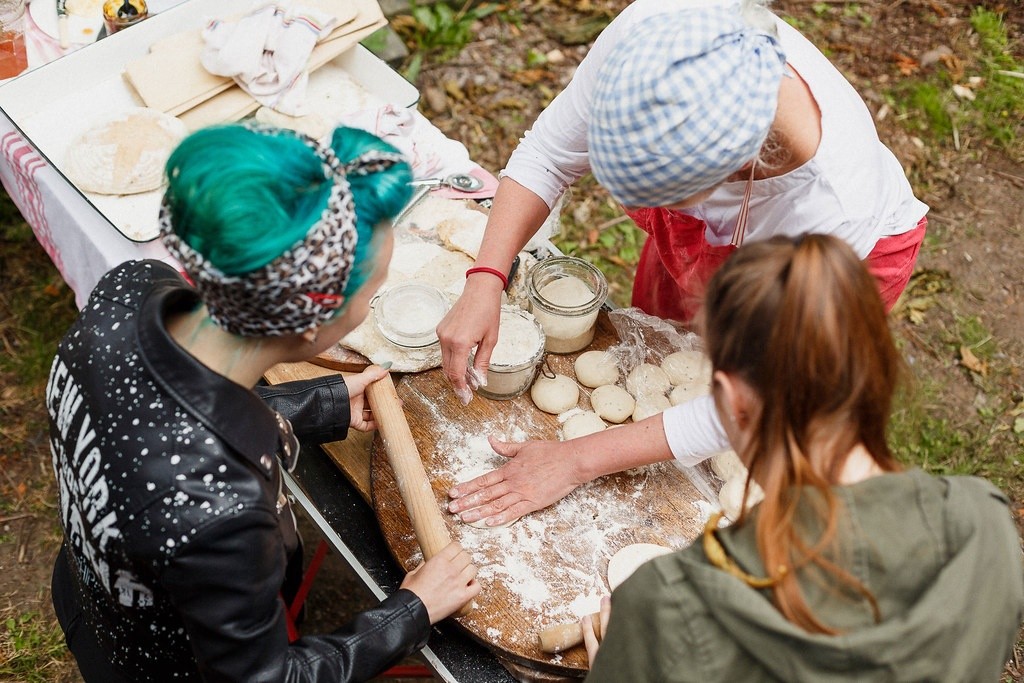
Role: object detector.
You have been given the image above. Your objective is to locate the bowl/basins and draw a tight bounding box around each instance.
[374,280,454,349]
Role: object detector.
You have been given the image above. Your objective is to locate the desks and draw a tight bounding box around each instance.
[263,198,618,683]
[0,0,482,311]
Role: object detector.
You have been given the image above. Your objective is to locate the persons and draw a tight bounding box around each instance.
[45,125,478,683]
[436,0,928,531]
[579,233,1024,683]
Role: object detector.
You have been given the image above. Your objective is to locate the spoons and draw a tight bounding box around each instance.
[412,173,482,193]
[117,0,138,22]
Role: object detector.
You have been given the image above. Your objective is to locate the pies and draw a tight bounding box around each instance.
[63,105,189,196]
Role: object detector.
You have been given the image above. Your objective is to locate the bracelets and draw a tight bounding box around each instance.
[466,268,509,290]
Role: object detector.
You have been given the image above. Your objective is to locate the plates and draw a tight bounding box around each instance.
[29,0,105,39]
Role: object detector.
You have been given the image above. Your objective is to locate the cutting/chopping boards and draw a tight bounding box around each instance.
[308,198,538,373]
[374,311,734,671]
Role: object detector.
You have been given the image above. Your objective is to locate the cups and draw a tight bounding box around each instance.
[0,0,28,80]
[101,0,148,37]
[524,256,608,354]
[463,305,546,401]
